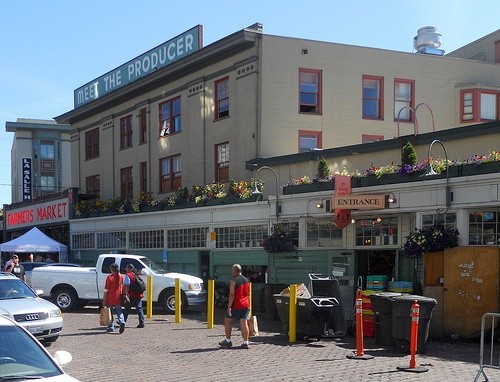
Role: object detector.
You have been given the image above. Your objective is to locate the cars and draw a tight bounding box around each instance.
[15,262,83,279]
[0,271,65,345]
[1,315,81,382]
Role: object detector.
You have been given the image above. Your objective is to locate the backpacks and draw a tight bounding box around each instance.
[132,272,146,294]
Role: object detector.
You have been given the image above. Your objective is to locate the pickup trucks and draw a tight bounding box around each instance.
[30,254,208,313]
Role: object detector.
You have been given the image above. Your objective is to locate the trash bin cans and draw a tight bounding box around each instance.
[308,272,347,338]
[272,294,341,341]
[369,293,411,347]
[389,294,437,353]
[264,284,289,321]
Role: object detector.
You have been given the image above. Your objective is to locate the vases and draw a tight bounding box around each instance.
[283,160,500,196]
[74,193,263,218]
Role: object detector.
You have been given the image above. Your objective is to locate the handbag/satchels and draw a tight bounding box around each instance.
[100,306,112,326]
[247,315,258,336]
[121,293,131,307]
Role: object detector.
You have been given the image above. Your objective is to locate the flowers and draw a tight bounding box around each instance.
[400,223,460,257]
[286,150,500,186]
[167,177,264,205]
[71,177,159,219]
[261,222,294,253]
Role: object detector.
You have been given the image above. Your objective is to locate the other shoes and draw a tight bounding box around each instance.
[119,323,125,333]
[116,320,120,324]
[219,340,232,347]
[241,344,249,349]
[137,324,145,328]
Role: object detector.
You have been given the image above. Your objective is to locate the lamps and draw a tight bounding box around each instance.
[419,140,450,214]
[387,198,396,203]
[302,49,307,55]
[252,166,281,223]
[316,204,323,208]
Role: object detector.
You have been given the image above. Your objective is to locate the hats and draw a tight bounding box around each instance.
[11,255,18,259]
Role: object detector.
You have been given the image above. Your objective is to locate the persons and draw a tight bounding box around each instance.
[101,263,125,334]
[116,263,145,328]
[5,254,26,283]
[5,253,14,272]
[219,264,250,349]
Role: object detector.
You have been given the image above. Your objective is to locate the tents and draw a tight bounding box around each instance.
[0,226,68,271]
